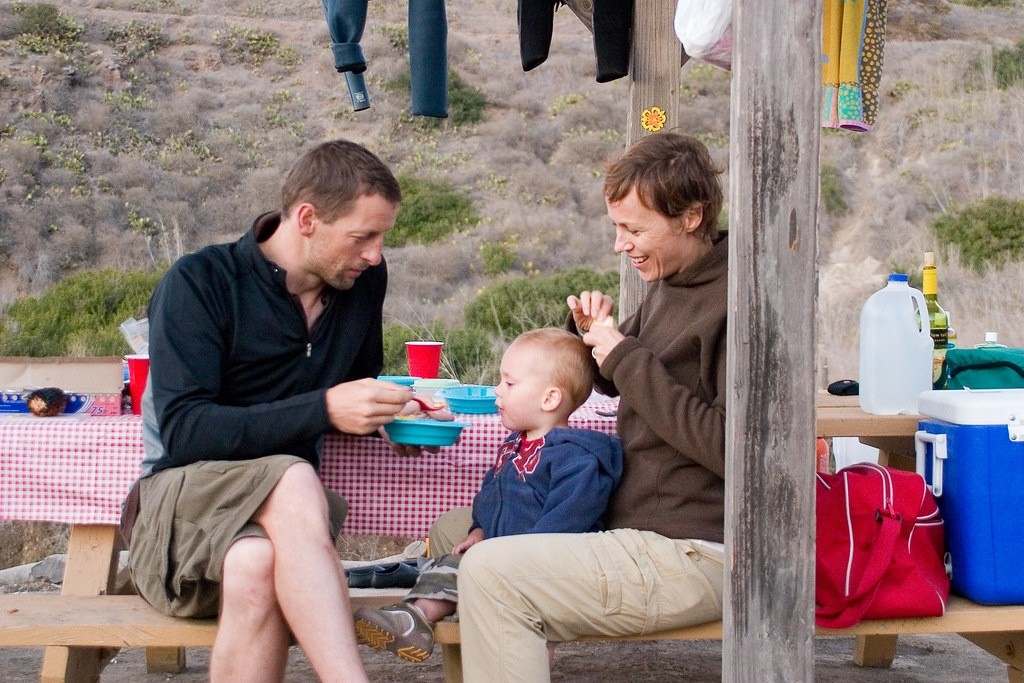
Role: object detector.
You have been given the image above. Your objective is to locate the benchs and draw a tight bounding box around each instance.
[813,592,1024,638]
[0,594,730,648]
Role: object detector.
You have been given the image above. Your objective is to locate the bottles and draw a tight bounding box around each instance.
[973,332,1009,349]
[858,274,935,415]
[923,252,949,390]
[943,310,957,350]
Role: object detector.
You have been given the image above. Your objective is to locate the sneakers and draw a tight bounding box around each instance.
[353,604,436,662]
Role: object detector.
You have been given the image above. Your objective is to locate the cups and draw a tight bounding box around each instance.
[404,341,444,378]
[127,354,151,416]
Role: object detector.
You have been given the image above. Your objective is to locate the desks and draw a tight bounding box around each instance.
[0,397,620,683]
[813,391,1024,682]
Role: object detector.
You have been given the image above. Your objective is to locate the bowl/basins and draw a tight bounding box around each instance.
[384,419,465,446]
[377,374,422,385]
[439,384,498,414]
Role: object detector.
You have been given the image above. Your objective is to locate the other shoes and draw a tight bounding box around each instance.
[348,563,386,588]
[371,562,420,588]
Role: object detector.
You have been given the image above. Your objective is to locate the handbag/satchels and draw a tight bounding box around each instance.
[814,465,949,632]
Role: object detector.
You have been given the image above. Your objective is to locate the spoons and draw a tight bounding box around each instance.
[411,396,445,412]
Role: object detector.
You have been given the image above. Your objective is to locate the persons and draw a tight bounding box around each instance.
[353,326,625,661]
[116,138,452,683]
[432,130,726,680]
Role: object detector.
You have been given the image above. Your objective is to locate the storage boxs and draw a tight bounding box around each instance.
[0,391,123,416]
[914,391,1024,607]
